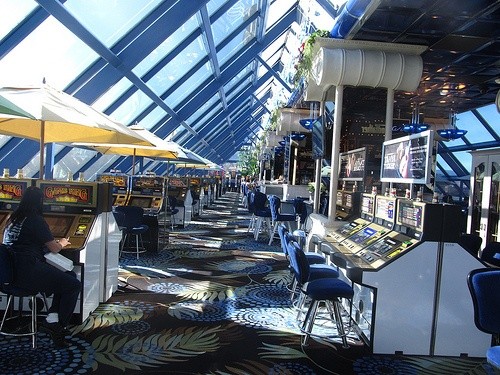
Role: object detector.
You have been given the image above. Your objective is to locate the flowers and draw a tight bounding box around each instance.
[297,29,330,78]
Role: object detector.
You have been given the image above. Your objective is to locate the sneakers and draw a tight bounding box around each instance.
[37,318,72,336]
[49,333,66,346]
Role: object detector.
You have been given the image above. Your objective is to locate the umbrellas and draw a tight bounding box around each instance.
[0,76,225,180]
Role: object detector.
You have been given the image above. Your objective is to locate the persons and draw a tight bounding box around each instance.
[2,186,81,346]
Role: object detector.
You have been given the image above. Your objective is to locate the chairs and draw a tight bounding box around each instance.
[241,184,306,246]
[191,191,197,218]
[466,266,500,369]
[115,206,149,260]
[0,243,49,349]
[278,225,355,352]
[164,197,178,230]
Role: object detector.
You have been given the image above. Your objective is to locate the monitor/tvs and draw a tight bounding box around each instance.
[129,198,152,208]
[0,212,9,224]
[168,189,179,197]
[43,214,76,238]
[380,130,434,184]
[338,147,367,181]
